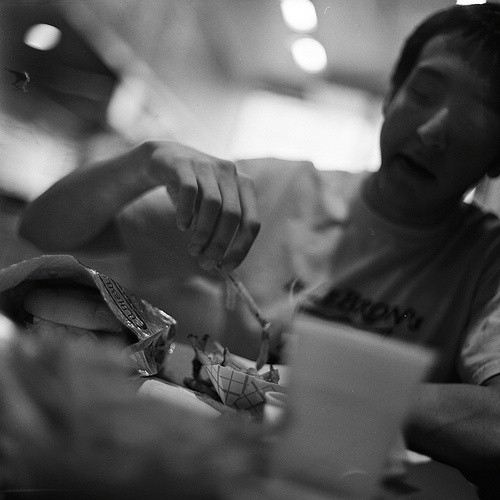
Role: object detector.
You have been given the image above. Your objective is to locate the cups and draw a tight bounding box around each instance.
[273,308,436,497]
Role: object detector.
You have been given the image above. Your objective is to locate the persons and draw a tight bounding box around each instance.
[19,0,500,500]
